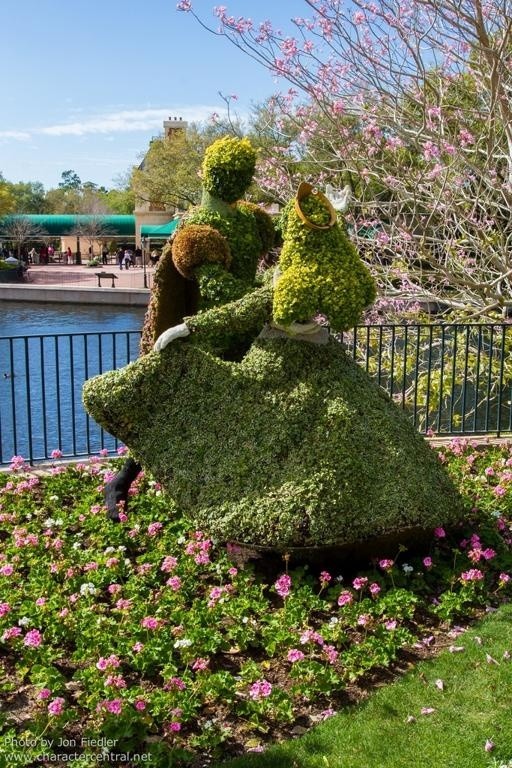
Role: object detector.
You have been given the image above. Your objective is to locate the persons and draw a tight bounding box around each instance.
[27,239,161,272]
